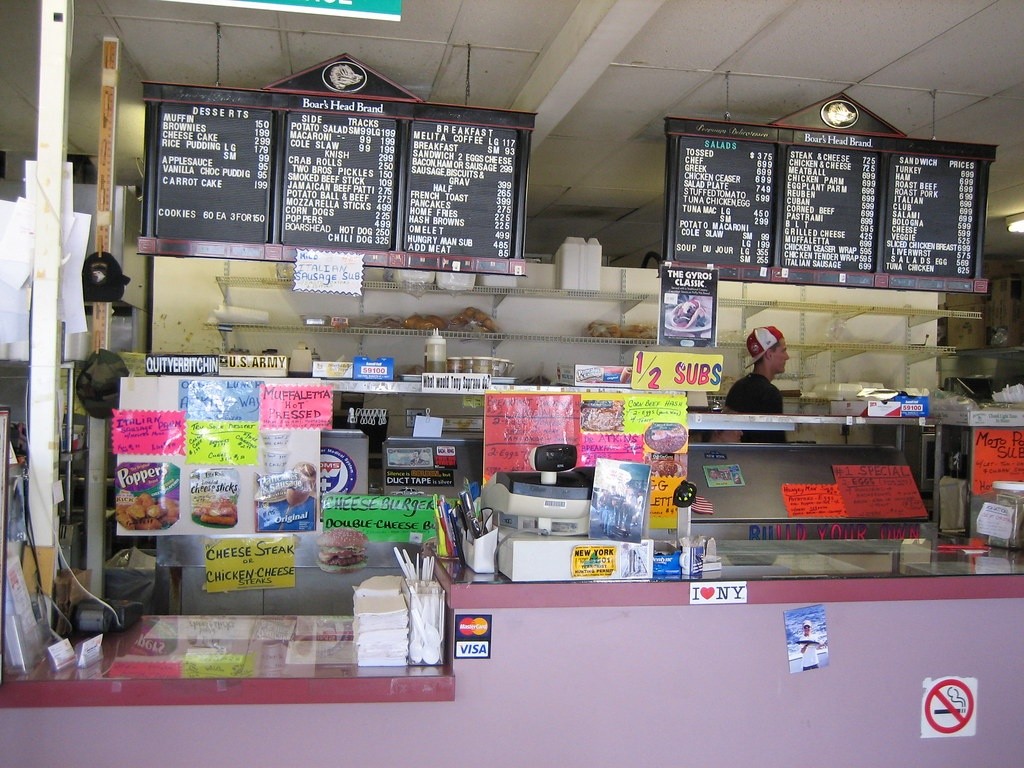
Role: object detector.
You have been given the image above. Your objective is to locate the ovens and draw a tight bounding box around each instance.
[874,424,953,493]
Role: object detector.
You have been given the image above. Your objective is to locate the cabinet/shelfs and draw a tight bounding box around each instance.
[205,275,984,368]
[0,360,90,524]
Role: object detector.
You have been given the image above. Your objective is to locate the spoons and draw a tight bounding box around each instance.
[392,547,445,665]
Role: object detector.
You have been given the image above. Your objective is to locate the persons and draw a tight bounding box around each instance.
[708,326,789,444]
[734,475,741,484]
[598,486,644,538]
[720,471,730,480]
[709,469,717,479]
[732,466,736,472]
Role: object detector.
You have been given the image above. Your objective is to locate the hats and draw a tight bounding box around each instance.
[76,348,130,420]
[744,326,782,368]
[83,252,130,302]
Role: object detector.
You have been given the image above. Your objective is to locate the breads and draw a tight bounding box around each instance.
[588,321,657,340]
[403,307,501,333]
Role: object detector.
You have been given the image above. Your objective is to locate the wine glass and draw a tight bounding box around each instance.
[278,463,316,530]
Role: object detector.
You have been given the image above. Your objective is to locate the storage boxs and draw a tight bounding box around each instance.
[464,523,499,573]
[703,562,722,572]
[831,401,902,417]
[653,542,704,575]
[402,573,446,666]
[887,393,930,418]
[353,355,394,381]
[218,354,289,377]
[557,362,634,388]
[313,361,353,378]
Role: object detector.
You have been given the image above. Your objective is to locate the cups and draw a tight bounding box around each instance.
[263,430,291,474]
[447,356,514,377]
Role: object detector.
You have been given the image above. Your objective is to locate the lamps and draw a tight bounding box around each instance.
[1006,212,1024,233]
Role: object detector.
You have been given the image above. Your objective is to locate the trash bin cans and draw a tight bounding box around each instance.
[103,548,157,616]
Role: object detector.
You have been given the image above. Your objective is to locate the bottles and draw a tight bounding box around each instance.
[424,328,447,373]
[985,480,1024,550]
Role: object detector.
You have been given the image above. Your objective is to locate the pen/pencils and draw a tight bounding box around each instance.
[431,476,481,567]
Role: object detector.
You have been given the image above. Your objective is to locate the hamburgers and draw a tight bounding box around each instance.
[314,527,369,573]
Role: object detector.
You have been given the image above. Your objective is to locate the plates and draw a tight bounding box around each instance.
[491,376,519,385]
[398,373,423,381]
[664,311,712,332]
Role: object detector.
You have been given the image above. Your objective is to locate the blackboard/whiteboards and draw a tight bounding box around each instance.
[659,116,998,297]
[148,80,535,269]
